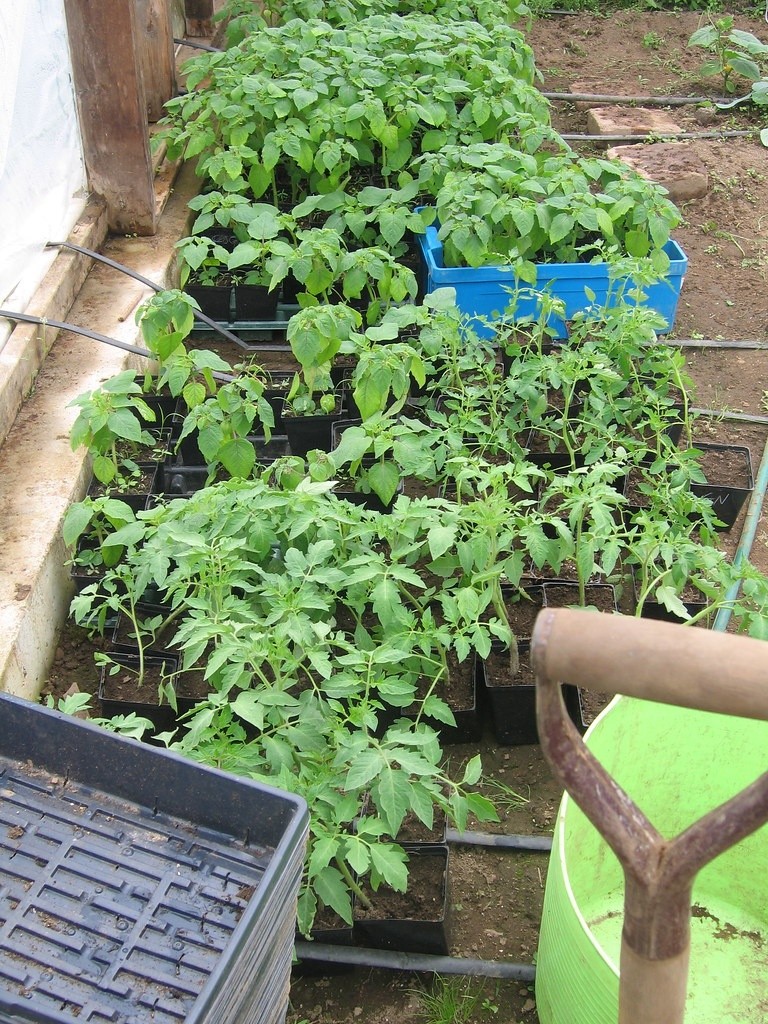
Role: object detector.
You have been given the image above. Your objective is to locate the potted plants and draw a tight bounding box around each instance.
[64,0,768,958]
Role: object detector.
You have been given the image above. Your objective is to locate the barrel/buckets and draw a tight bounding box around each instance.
[538,692,768,1024]
